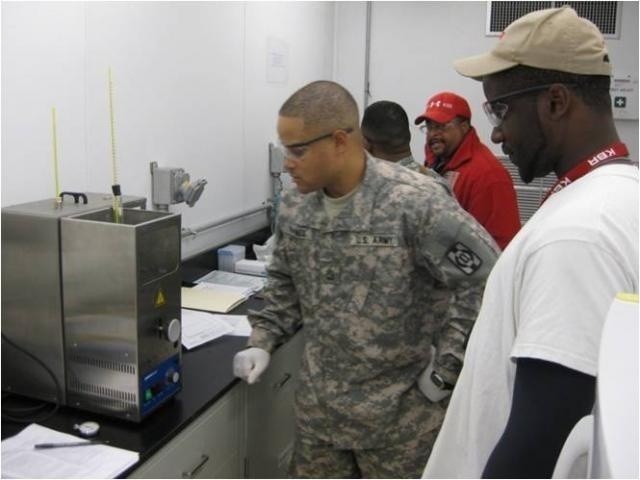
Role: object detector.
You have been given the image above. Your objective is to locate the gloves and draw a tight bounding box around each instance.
[417,361,451,403]
[233,347,271,386]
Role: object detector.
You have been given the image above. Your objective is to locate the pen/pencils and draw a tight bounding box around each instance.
[35,439,111,449]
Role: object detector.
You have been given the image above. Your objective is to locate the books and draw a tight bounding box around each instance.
[1,423,140,479]
[175,287,247,312]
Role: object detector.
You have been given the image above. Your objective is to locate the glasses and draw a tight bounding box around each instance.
[281,128,355,162]
[481,83,579,128]
[414,91,472,125]
[420,121,465,134]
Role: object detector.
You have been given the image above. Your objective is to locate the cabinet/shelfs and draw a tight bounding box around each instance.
[124,333,303,479]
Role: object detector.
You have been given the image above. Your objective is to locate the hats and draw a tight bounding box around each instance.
[455,3,614,78]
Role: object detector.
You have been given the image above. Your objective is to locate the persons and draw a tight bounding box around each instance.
[415,92,520,250]
[235,82,503,477]
[360,101,443,179]
[423,7,639,478]
